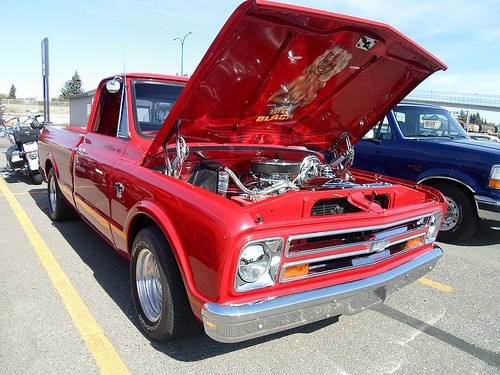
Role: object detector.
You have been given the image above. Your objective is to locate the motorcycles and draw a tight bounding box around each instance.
[0,114,46,186]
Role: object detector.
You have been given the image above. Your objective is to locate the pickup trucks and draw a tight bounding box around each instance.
[38,0,448,345]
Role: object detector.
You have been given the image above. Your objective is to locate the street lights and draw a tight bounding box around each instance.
[172,32,193,75]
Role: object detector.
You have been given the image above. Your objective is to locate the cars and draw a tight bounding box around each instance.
[344,101,500,244]
